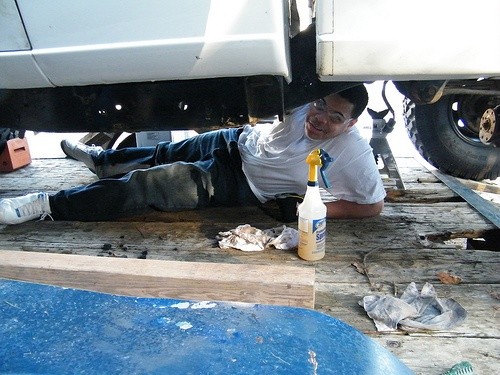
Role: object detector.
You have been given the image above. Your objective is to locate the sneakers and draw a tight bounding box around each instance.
[0,193,54,225]
[61,139,103,174]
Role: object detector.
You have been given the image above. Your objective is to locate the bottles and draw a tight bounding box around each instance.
[298,149,334,261]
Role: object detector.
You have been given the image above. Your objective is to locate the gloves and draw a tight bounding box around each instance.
[268,194,303,222]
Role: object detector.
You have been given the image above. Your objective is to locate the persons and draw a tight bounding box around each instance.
[0,82,387,224]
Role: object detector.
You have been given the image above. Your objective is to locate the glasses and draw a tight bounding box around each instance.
[313,98,345,122]
